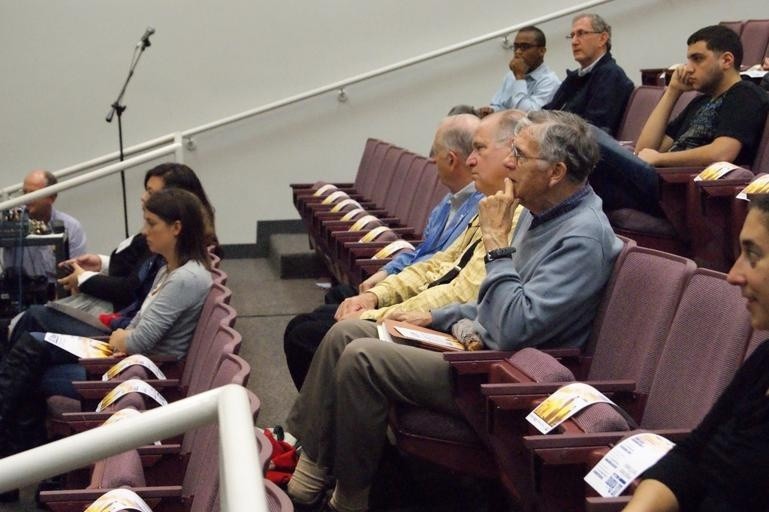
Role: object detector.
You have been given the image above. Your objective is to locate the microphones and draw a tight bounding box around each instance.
[138,28,154,46]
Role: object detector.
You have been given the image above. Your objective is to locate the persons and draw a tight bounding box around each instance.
[448,25,562,120]
[282,104,622,512]
[583,24,768,213]
[539,14,634,141]
[0,162,219,461]
[624,175,768,512]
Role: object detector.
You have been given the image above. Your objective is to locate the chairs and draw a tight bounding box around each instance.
[44,243,295,512]
[290,19,769,512]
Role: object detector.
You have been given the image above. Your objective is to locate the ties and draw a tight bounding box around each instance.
[427,238,481,289]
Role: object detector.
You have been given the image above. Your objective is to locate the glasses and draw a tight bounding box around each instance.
[566,29,601,38]
[509,44,540,51]
[511,139,547,160]
